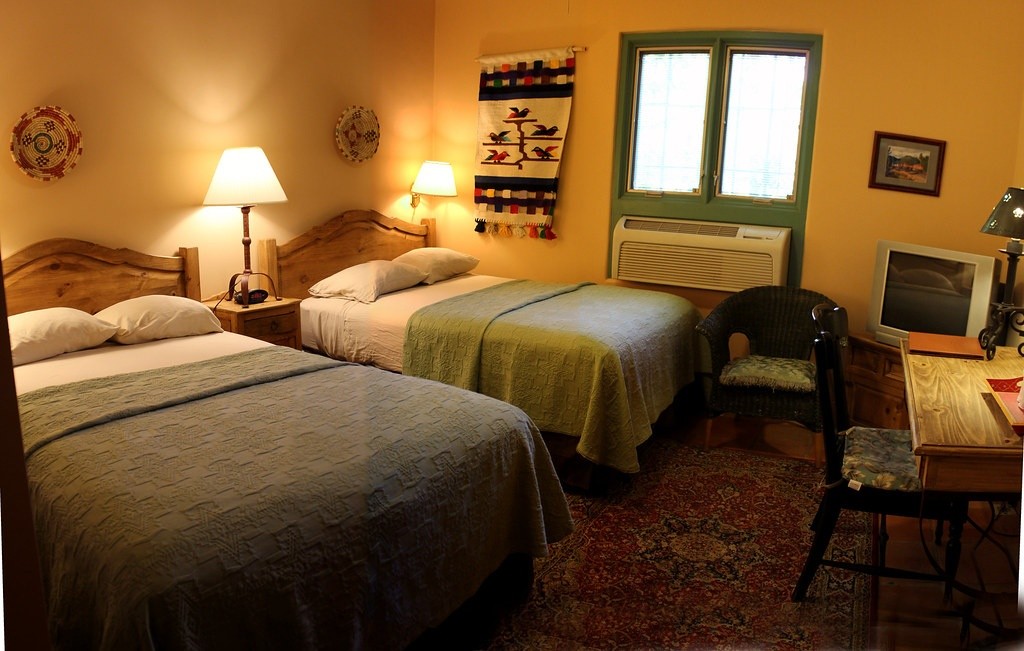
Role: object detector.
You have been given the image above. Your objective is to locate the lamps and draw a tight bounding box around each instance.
[202,146,288,310]
[409,160,459,210]
[979,187,1024,360]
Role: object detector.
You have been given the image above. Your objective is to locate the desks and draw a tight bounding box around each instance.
[845,332,911,430]
[898,338,1024,651]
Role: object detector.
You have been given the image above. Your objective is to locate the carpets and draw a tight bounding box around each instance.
[483,442,879,651]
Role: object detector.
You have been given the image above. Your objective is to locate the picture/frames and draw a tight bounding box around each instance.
[867,130,947,199]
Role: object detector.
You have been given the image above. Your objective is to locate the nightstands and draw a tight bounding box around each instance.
[201,291,303,351]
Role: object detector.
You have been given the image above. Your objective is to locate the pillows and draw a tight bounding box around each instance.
[392,247,481,285]
[307,259,431,305]
[8,306,121,368]
[94,295,224,346]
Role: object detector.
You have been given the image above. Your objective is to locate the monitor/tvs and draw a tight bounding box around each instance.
[865,240,1002,348]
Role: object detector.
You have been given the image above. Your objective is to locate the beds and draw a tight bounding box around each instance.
[258,209,711,475]
[2,238,574,651]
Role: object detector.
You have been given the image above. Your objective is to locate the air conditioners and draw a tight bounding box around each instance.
[611,215,791,294]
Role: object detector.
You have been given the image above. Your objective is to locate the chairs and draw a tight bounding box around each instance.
[791,305,969,607]
[696,286,848,433]
